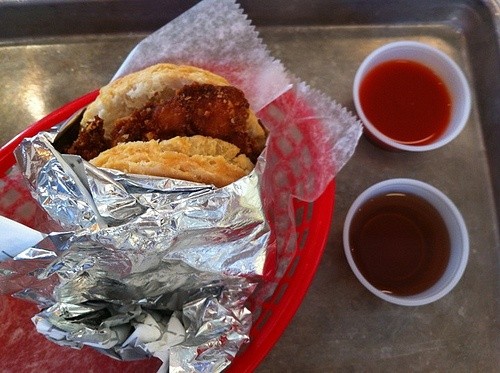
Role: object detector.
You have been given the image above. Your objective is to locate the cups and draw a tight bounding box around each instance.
[343,178,470,307]
[353,41,471,154]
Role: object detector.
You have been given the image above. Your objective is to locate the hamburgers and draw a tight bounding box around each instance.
[64,63,266,189]
